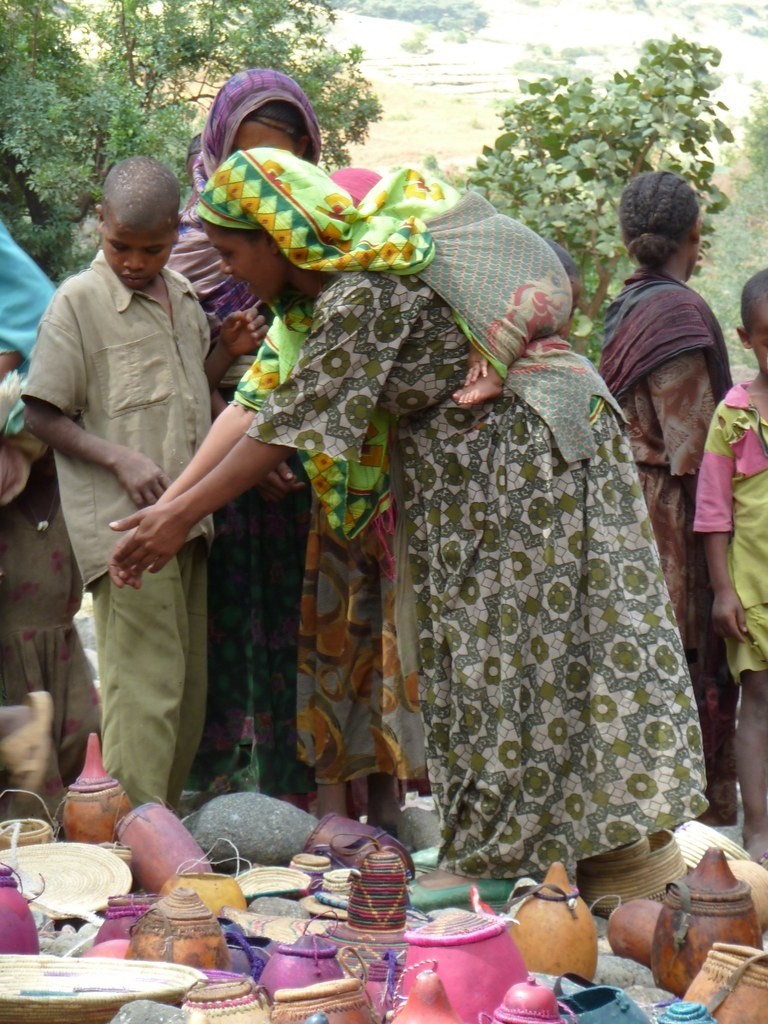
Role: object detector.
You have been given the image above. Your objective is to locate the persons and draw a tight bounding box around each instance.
[1,68,768,881]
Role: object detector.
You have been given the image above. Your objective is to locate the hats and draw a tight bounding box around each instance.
[300,868,361,919]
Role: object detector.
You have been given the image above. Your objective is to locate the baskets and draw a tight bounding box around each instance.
[0,954,209,1024]
[0,843,132,919]
[235,867,311,903]
